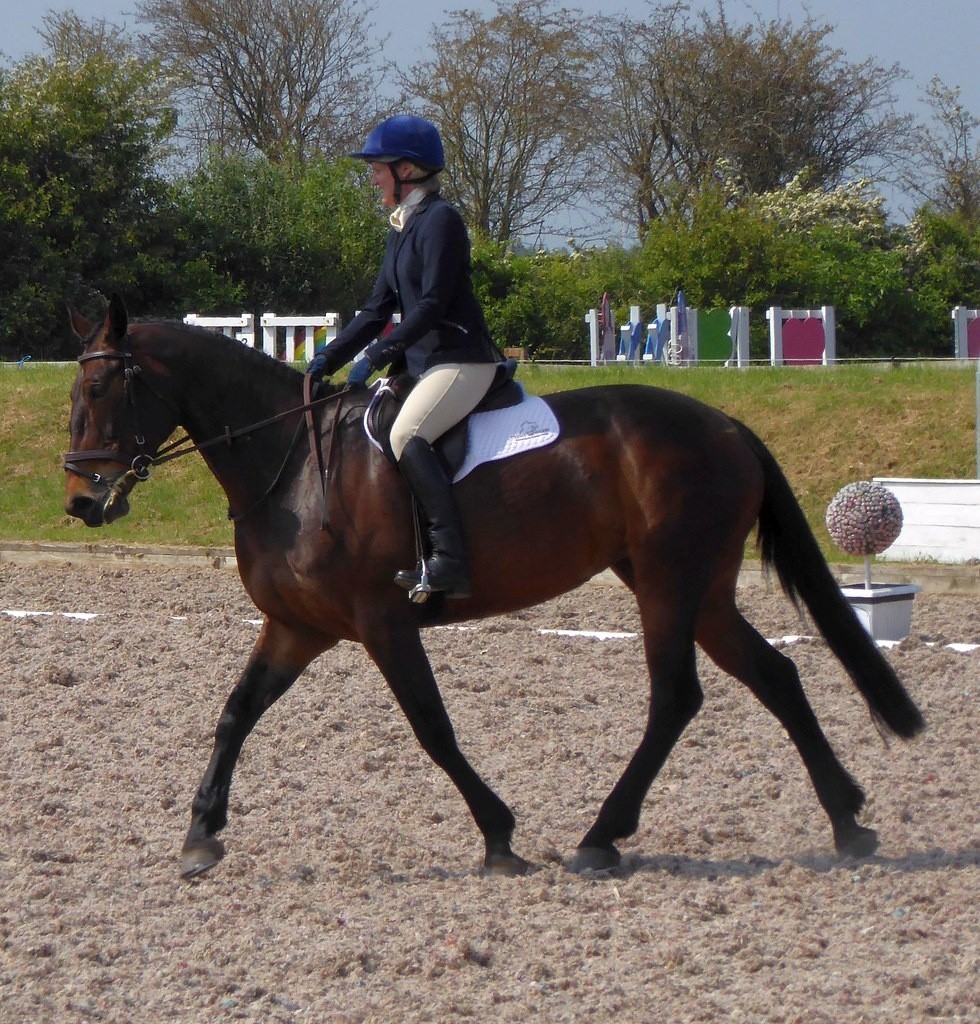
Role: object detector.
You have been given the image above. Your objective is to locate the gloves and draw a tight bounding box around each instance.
[347,358,375,384]
[307,354,328,382]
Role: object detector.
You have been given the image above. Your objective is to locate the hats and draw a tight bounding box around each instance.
[349,114,448,170]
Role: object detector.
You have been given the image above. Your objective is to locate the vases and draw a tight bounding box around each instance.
[839,582,921,642]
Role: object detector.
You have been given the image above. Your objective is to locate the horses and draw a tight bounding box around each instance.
[63,292,925,882]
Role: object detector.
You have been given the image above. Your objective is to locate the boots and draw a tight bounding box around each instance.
[394,436,472,599]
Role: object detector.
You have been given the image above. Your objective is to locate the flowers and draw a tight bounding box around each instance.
[825,481,903,555]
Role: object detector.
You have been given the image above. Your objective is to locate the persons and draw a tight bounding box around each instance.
[306,114,508,599]
